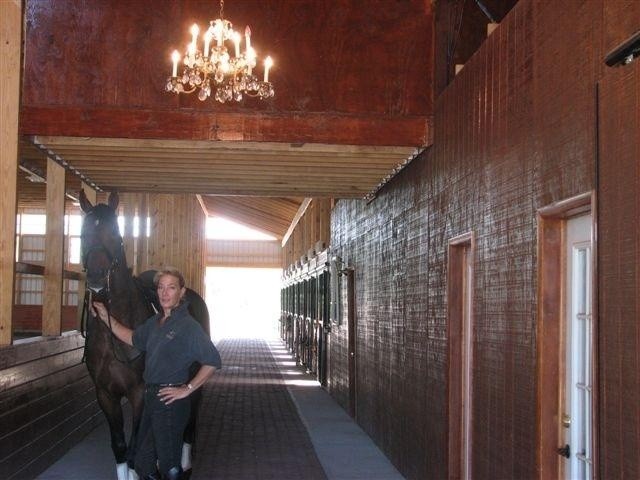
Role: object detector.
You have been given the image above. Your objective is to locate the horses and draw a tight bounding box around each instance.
[78,187,211,480]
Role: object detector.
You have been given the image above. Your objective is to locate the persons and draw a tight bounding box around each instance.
[88,264,222,479]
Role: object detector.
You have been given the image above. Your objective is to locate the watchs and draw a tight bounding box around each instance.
[186,382,195,392]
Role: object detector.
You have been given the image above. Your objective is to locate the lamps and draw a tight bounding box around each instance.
[159,0,276,106]
